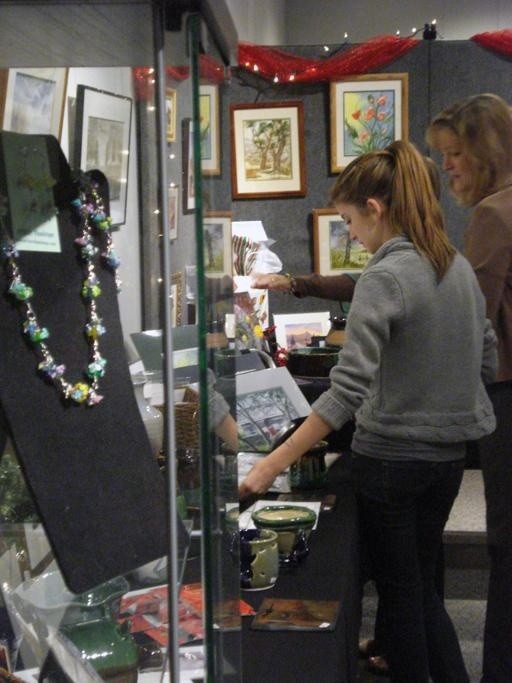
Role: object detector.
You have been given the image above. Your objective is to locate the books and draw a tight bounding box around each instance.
[250,597,341,633]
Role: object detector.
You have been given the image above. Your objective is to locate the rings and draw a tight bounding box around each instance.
[271,277,274,281]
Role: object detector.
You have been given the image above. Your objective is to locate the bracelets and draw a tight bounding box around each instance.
[284,272,296,296]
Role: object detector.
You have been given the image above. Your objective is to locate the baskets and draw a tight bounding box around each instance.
[154,401,200,463]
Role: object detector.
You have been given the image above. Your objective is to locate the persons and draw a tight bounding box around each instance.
[250,270,390,676]
[423,92,511,682]
[237,140,497,683]
[207,367,237,450]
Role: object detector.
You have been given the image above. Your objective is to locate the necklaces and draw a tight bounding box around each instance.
[1,168,113,407]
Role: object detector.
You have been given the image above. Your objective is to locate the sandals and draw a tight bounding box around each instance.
[358,640,389,673]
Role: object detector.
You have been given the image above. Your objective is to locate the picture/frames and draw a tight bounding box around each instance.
[189,367,315,457]
[161,85,185,329]
[192,78,236,279]
[272,310,332,358]
[0,65,133,230]
[228,72,409,277]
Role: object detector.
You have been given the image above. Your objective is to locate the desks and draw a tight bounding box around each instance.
[178,478,365,681]
